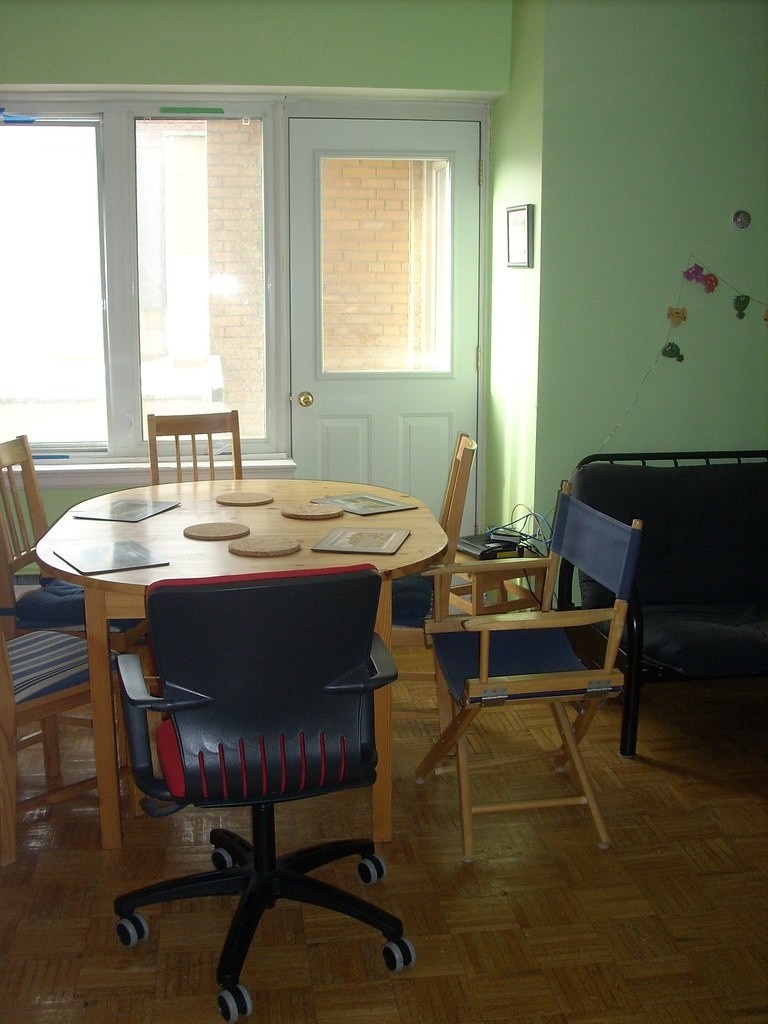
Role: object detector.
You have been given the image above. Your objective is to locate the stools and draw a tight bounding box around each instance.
[449,533,546,613]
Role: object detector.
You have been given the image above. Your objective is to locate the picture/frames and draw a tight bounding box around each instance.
[310,526,413,554]
[54,539,170,575]
[309,493,419,516]
[505,203,534,268]
[73,497,182,523]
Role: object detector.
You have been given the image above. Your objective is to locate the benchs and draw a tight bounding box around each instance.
[557,450,768,758]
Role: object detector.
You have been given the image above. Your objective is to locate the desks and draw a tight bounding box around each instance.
[35,478,448,843]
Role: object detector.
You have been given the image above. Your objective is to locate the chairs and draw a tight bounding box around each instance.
[0,435,166,730]
[146,411,244,485]
[0,629,146,865]
[413,481,644,863]
[112,563,414,1024]
[392,430,477,757]
